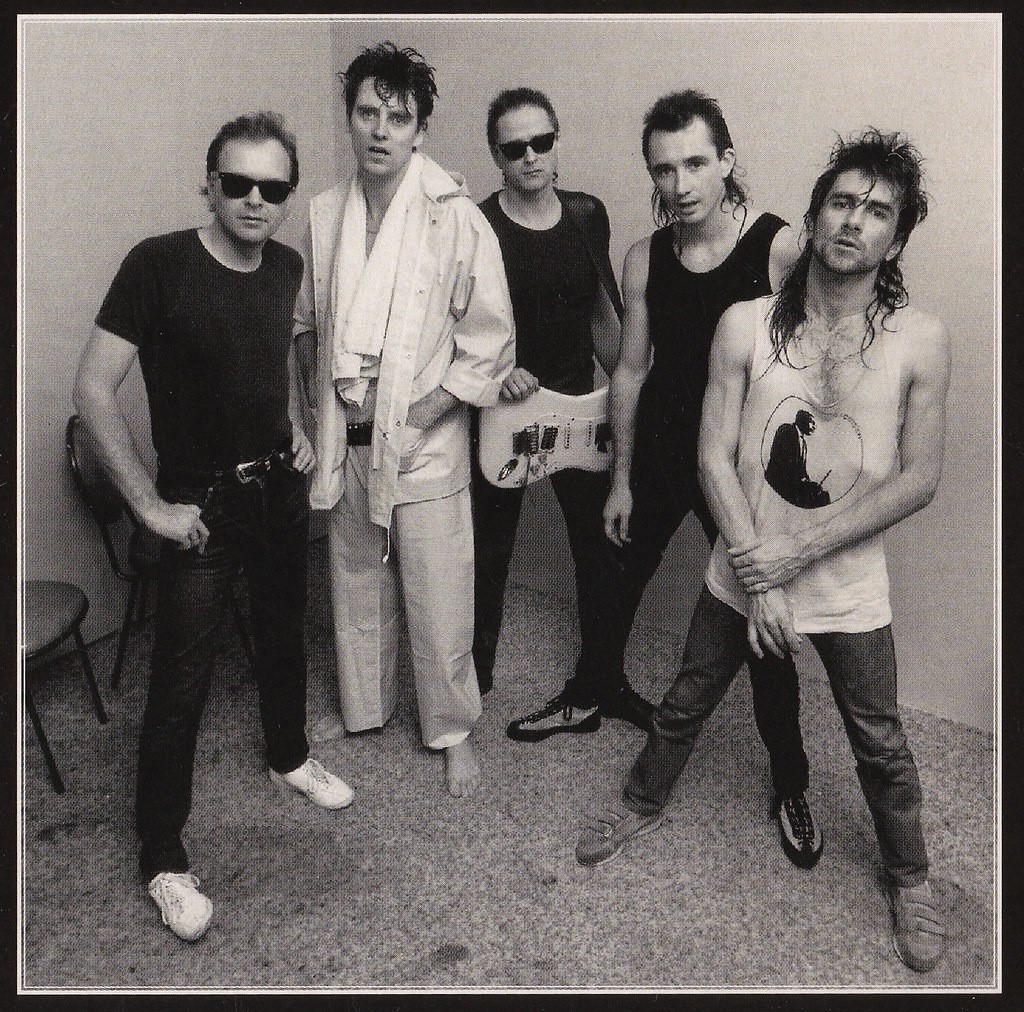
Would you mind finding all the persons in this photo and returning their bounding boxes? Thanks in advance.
[507,88,823,870]
[293,44,517,800]
[575,137,944,972]
[470,88,660,731]
[71,114,355,941]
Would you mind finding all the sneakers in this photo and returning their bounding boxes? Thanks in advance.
[268,764,355,810]
[148,870,213,940]
[883,880,944,973]
[772,793,823,868]
[576,798,662,866]
[507,697,601,742]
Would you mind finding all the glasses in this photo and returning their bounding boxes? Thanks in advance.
[218,171,293,204]
[498,131,557,162]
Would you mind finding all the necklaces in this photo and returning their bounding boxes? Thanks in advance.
[794,363,866,408]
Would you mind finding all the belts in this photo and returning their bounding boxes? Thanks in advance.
[345,422,373,446]
[161,441,294,485]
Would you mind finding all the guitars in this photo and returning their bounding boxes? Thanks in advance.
[476,385,616,489]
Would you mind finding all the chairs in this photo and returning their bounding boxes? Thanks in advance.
[23,578,109,793]
[67,414,256,690]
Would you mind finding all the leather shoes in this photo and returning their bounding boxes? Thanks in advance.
[601,685,659,732]
[478,673,494,696]
